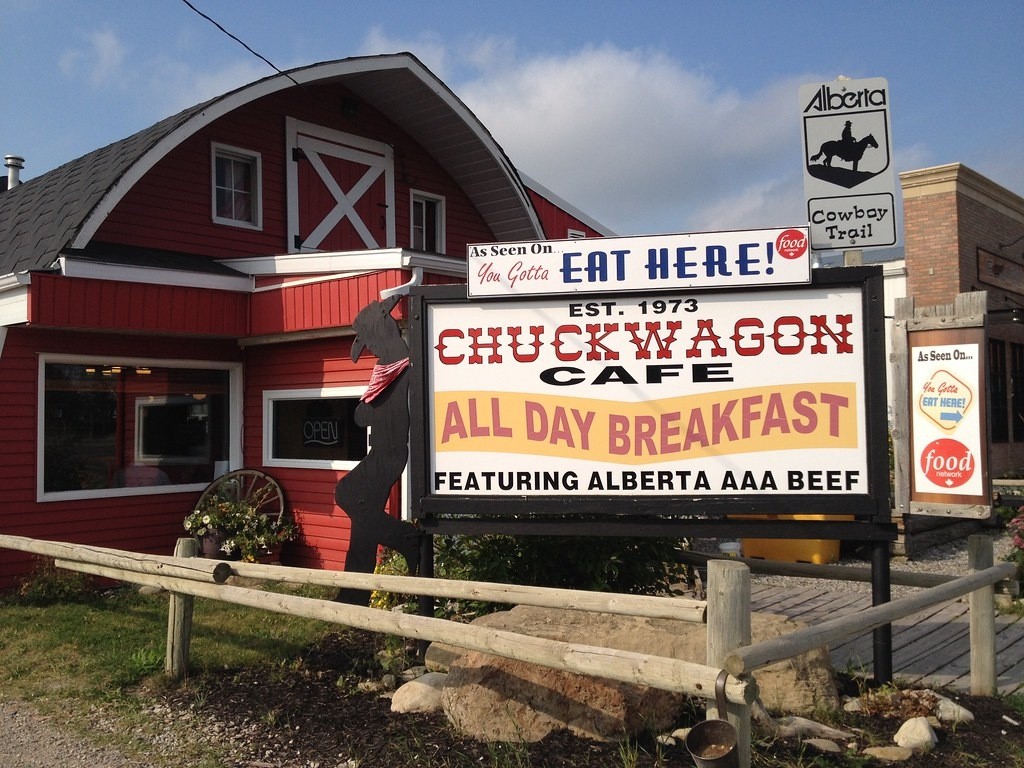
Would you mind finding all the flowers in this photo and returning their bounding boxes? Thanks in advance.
[182,471,300,564]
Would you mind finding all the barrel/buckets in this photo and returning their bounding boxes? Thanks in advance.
[685,720,739,768]
[719,542,741,558]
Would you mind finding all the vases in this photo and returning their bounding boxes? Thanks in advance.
[256,542,282,564]
[194,528,235,560]
